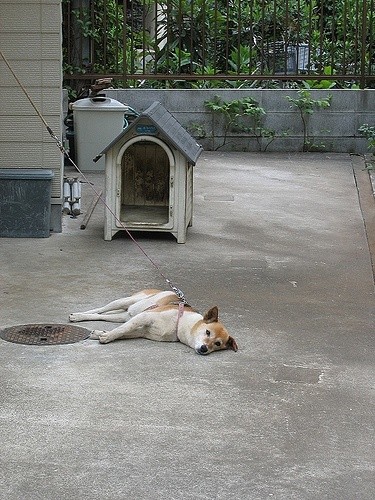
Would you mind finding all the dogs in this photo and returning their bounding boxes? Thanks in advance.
[68,289,238,356]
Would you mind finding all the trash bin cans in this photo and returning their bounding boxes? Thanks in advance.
[0,168,54,239]
[69,97,130,173]
